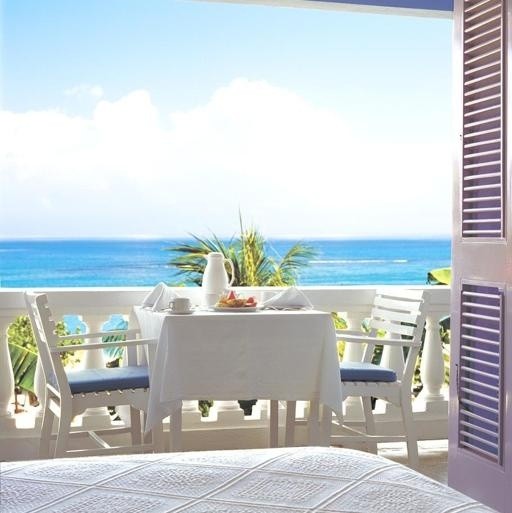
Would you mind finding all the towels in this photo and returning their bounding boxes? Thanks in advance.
[143,281,186,310]
[262,285,316,309]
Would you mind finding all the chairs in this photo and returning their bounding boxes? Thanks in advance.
[22,288,186,457]
[317,285,430,465]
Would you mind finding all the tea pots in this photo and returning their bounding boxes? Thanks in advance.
[201,252,235,306]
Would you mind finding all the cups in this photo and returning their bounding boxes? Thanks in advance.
[169,297,189,312]
[260,291,278,303]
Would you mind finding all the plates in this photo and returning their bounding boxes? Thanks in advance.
[209,304,264,312]
[164,309,196,315]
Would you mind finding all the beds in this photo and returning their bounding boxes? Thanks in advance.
[0,446,501,513]
